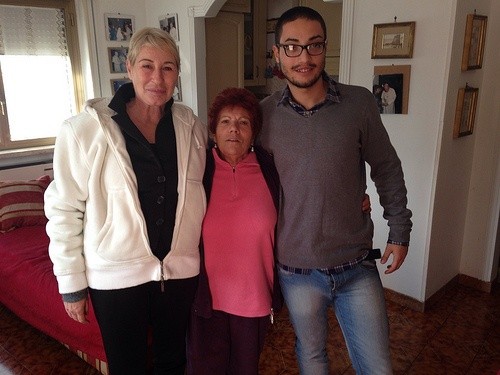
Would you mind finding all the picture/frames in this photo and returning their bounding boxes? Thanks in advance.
[103,11,135,42]
[110,77,131,96]
[108,47,130,73]
[370,22,415,59]
[371,65,415,115]
[453,86,479,139]
[461,13,489,72]
[159,14,183,102]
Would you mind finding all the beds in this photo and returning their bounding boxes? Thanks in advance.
[0,219,109,375]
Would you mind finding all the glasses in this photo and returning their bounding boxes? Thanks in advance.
[277,41,328,57]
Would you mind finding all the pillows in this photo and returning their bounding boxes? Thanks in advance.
[0,173,50,234]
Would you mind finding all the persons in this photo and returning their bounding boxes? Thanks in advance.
[106,17,183,106]
[183,89,374,374]
[254,6,413,374]
[371,79,397,113]
[42,26,211,375]
